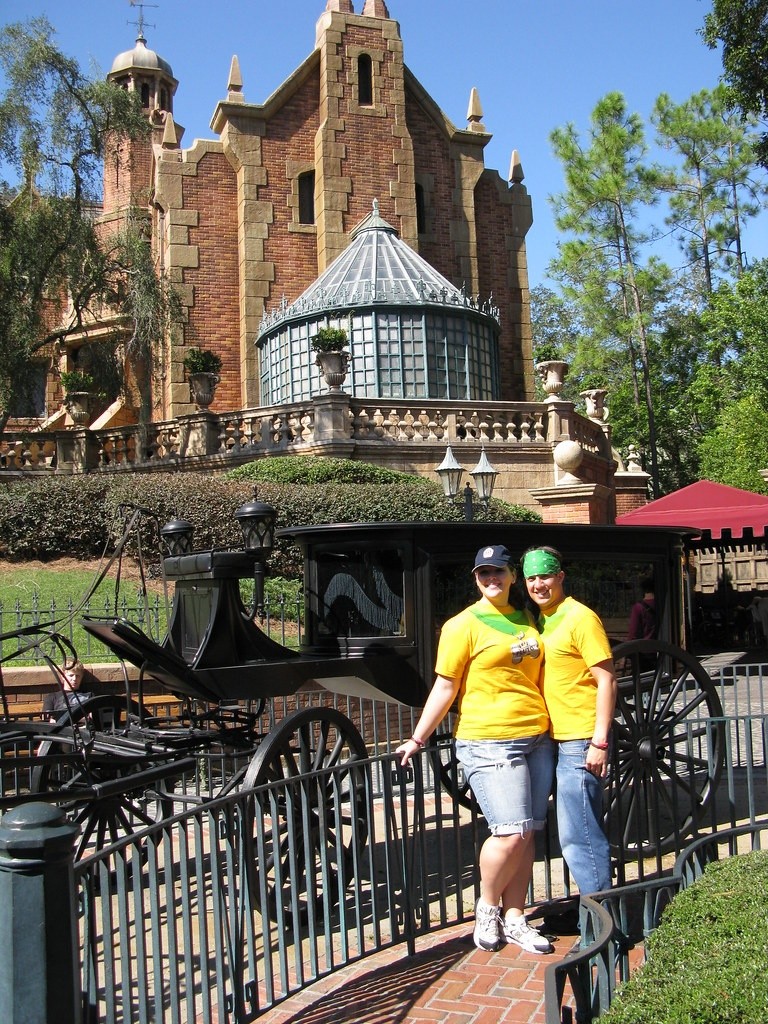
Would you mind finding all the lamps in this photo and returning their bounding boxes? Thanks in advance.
[156,485,277,625]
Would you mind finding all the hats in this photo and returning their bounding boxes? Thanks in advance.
[471,544,514,572]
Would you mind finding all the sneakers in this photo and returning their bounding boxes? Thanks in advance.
[473,896,503,952]
[498,917,555,954]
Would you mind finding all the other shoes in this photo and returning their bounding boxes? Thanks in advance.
[541,908,580,935]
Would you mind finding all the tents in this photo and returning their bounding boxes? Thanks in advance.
[615,480,768,539]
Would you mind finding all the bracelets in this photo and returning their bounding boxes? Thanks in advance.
[411,736,425,747]
[591,743,609,750]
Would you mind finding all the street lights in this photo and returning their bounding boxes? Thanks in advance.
[435,440,501,521]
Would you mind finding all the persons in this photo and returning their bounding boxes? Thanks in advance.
[628,579,657,670]
[397,545,556,953]
[42,659,95,754]
[747,597,761,623]
[519,546,617,895]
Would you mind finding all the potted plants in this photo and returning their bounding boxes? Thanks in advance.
[58,370,94,429]
[183,347,223,413]
[532,344,569,403]
[310,326,353,393]
[579,373,608,424]
